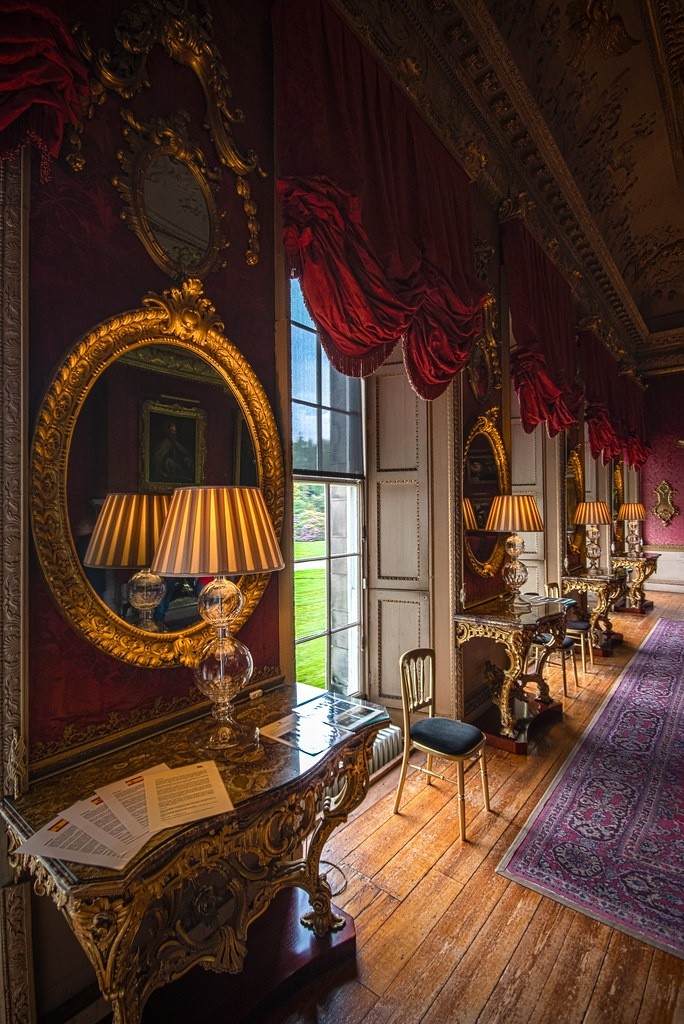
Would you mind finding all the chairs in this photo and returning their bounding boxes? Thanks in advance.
[394,649,490,841]
[524,582,594,696]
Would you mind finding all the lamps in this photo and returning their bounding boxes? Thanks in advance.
[572,502,611,572]
[84,492,174,633]
[486,495,545,607]
[151,486,285,761]
[617,503,647,555]
[463,498,478,529]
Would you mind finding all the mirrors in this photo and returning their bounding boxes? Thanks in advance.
[29,273,285,669]
[613,465,623,540]
[463,404,511,577]
[567,452,585,555]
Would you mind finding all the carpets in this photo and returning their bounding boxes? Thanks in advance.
[495,618,684,960]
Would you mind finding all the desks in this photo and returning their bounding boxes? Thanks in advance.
[454,553,663,738]
[0,682,392,1024]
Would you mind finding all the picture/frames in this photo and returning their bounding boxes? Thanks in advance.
[111,105,231,282]
[467,330,494,404]
[467,450,499,484]
[139,399,208,492]
